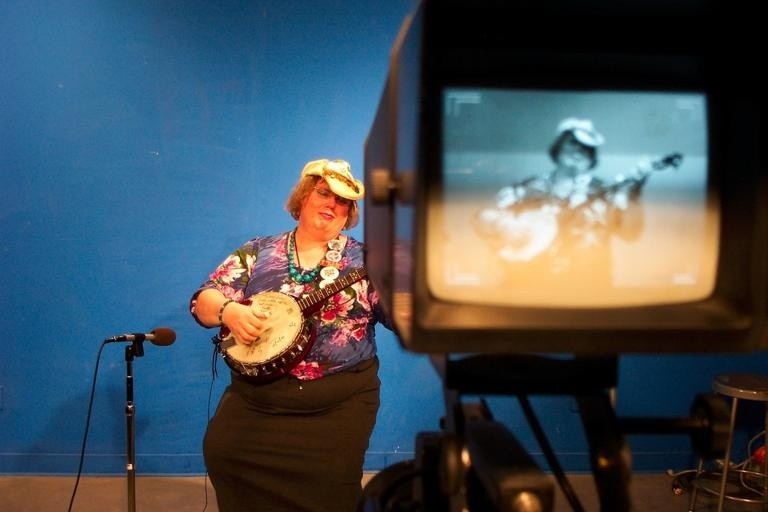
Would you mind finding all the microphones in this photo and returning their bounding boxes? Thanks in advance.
[106,327,176,345]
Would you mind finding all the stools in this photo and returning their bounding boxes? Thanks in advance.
[688,371,768,512]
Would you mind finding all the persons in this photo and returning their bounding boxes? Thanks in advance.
[481,118,645,278]
[190,159,392,512]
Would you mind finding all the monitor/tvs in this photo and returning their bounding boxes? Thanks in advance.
[425,85,724,310]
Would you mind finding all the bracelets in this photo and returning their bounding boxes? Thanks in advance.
[218,299,234,323]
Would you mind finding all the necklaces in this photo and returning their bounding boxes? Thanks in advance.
[288,227,321,284]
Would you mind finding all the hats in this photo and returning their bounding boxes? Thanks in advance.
[301,159,365,200]
[555,117,605,146]
